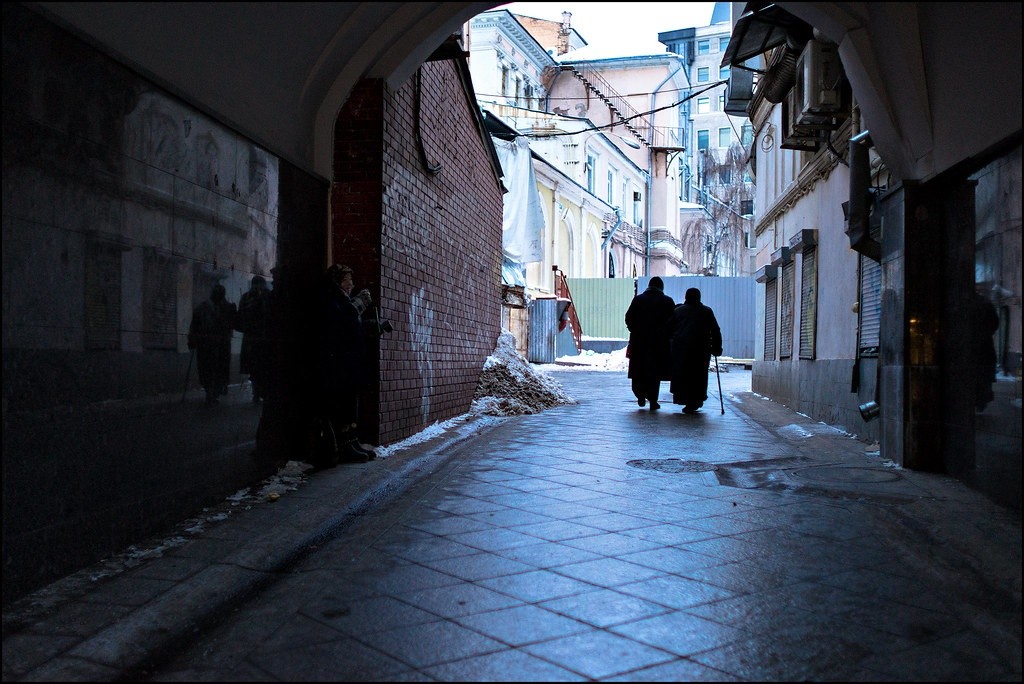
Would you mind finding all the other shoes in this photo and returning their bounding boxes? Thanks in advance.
[638,398,646,406]
[650,403,660,411]
[682,405,699,412]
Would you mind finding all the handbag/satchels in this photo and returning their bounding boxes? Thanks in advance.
[308,417,337,469]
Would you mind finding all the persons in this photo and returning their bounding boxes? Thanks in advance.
[187,277,274,405]
[317,262,377,462]
[625,276,722,413]
[919,261,999,473]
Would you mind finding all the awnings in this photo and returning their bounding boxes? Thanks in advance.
[720,1,811,73]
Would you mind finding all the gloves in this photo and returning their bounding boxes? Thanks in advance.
[357,288,372,306]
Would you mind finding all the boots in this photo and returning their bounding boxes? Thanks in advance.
[334,424,375,462]
